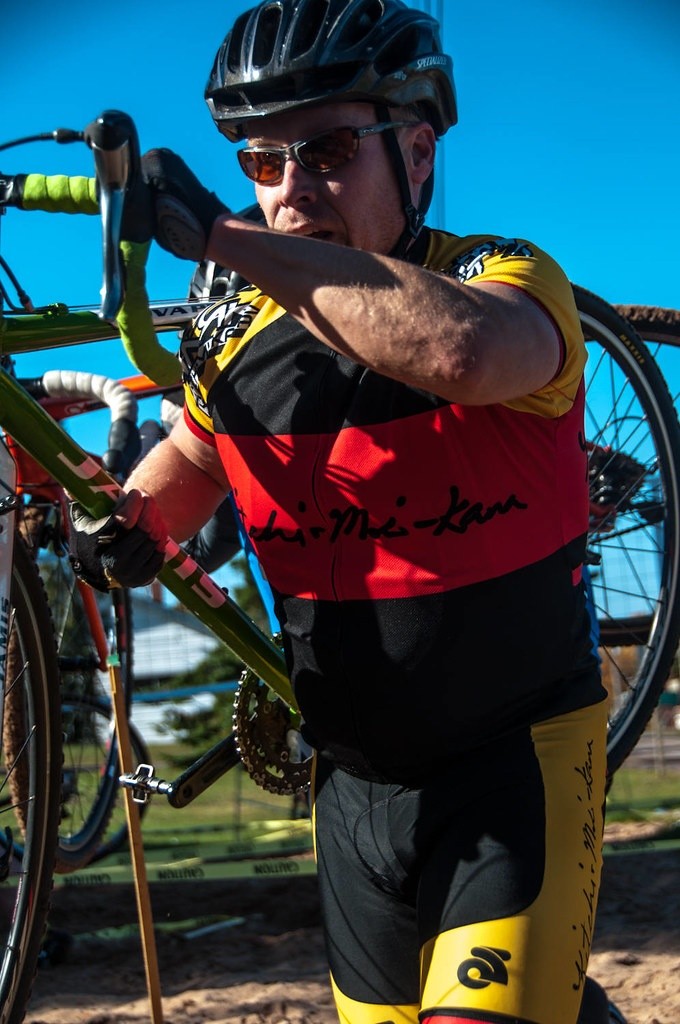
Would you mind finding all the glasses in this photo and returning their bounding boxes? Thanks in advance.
[236,120,421,185]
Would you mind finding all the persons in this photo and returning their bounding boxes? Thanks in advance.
[66,0,610,1024]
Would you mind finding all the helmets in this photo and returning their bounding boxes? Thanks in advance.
[205,0,458,136]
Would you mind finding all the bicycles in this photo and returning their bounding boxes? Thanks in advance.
[1,109,680,1024]
